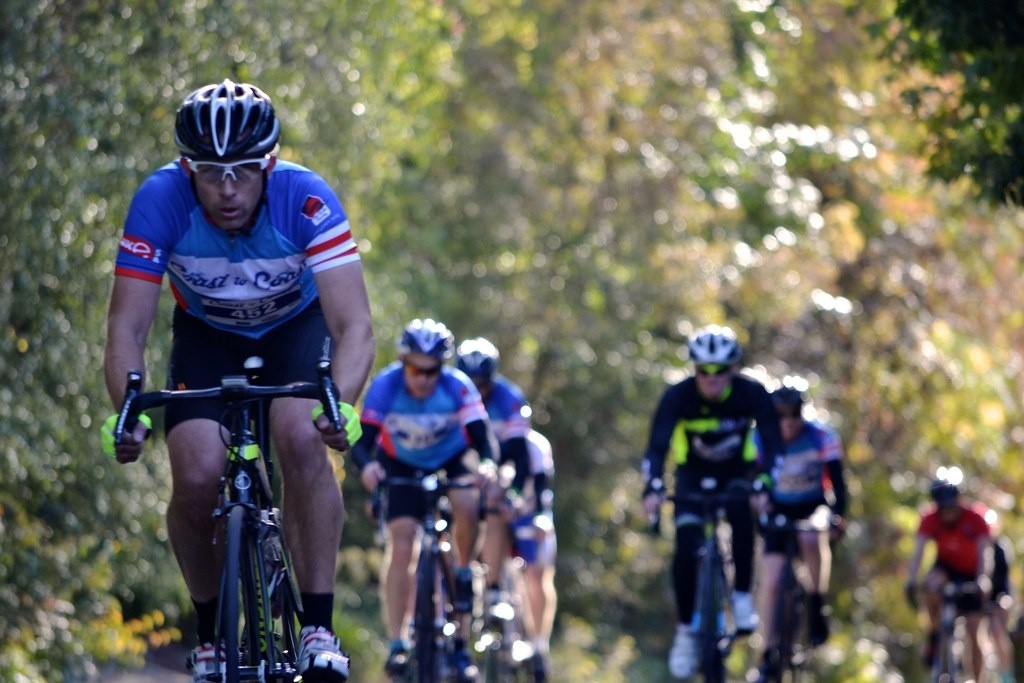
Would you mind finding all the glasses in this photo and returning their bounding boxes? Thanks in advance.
[185,155,270,186]
[403,357,442,378]
[696,364,731,377]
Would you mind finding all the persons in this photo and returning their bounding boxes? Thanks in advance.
[752,380,850,683]
[642,324,784,678]
[100,79,377,683]
[456,338,559,683]
[904,468,1016,683]
[351,318,501,683]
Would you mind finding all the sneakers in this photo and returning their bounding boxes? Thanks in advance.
[192,641,226,683]
[296,625,351,683]
[448,567,475,613]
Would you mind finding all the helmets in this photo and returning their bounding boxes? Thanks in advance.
[688,325,743,365]
[929,480,960,501]
[173,78,280,160]
[398,316,455,363]
[455,335,500,381]
[772,387,803,417]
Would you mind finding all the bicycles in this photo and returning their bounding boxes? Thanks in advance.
[372,468,533,683]
[646,480,767,683]
[757,507,837,683]
[114,356,342,683]
[910,578,993,683]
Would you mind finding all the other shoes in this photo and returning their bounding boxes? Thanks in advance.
[668,625,699,677]
[523,651,546,683]
[451,649,476,678]
[919,631,941,670]
[384,647,411,674]
[728,590,762,631]
[804,592,829,645]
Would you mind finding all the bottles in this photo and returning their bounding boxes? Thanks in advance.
[422,518,438,552]
[260,509,284,537]
